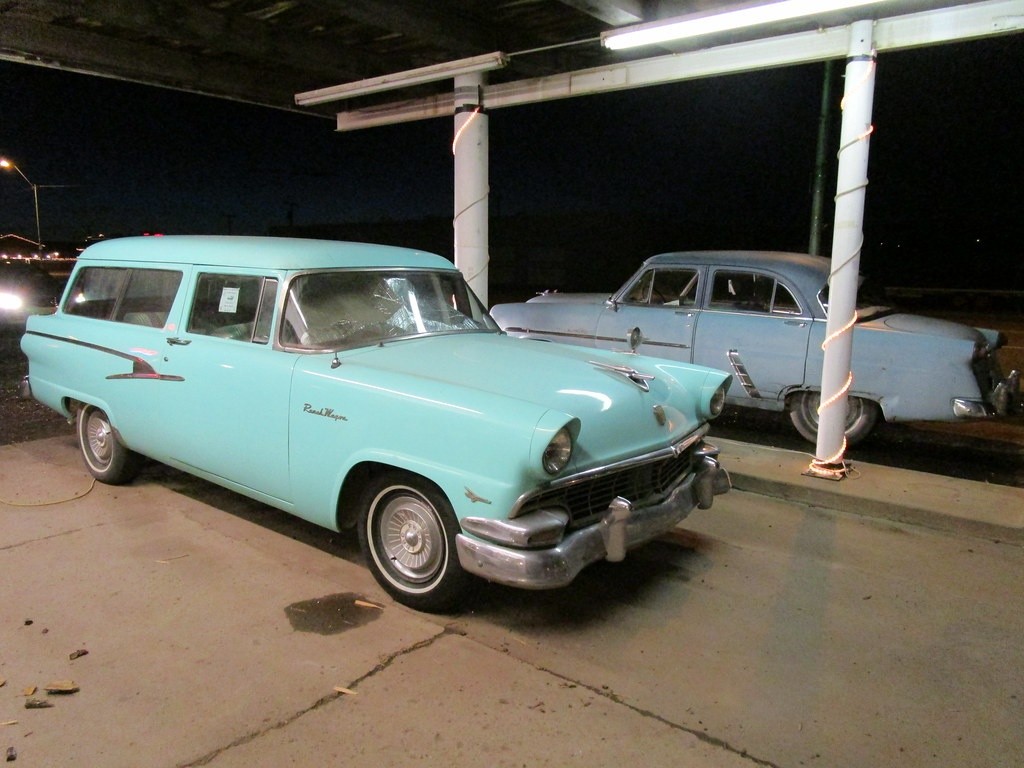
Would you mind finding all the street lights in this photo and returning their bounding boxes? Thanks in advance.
[1,159,45,275]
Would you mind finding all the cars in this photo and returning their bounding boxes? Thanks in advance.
[489,249,1021,446]
[0,261,88,334]
[19,235,734,613]
